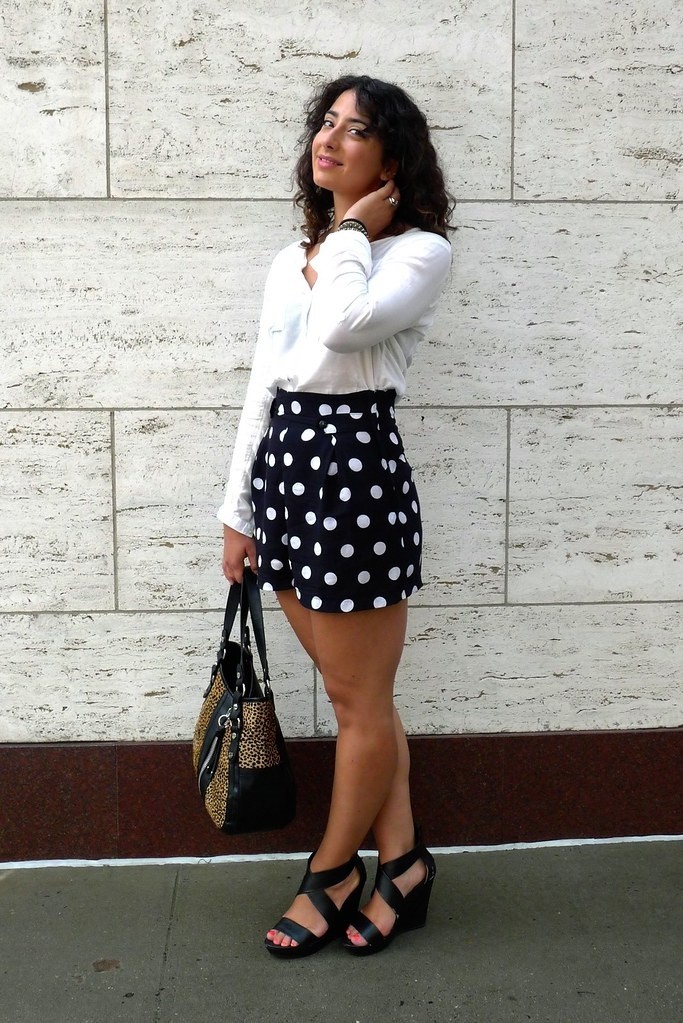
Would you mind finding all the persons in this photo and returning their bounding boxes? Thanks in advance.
[216,74,457,961]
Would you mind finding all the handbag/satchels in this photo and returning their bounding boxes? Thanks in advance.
[192,565,296,835]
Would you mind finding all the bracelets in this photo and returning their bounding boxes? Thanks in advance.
[337,218,371,239]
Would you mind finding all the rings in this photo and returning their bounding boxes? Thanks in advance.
[388,196,399,206]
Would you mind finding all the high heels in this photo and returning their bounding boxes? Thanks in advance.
[341,837,437,955]
[263,851,366,959]
